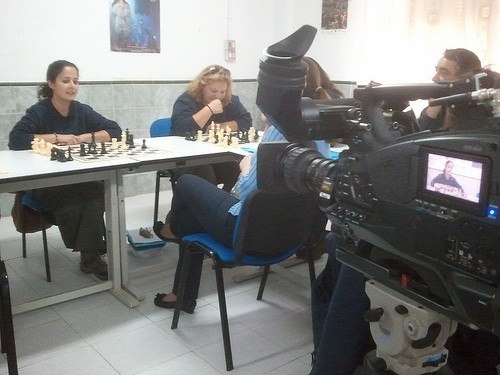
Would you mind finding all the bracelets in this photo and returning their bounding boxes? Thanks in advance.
[54,133,57,144]
[206,104,213,114]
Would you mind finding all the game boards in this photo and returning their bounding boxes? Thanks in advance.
[185,130,264,147]
[57,139,167,164]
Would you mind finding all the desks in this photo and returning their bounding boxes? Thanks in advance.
[229,140,349,290]
[0,128,264,315]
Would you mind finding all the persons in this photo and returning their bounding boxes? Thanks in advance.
[169,65,252,192]
[417,48,481,131]
[9,59,122,281]
[309,236,373,375]
[431,160,465,195]
[153,56,350,314]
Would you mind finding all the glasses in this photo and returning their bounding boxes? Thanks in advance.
[210,67,230,76]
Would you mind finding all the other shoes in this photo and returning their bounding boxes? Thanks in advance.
[74,234,107,254]
[153,221,180,243]
[154,293,196,314]
[81,255,108,280]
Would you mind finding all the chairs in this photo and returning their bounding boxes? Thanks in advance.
[150,118,179,224]
[20,190,106,282]
[170,140,319,371]
